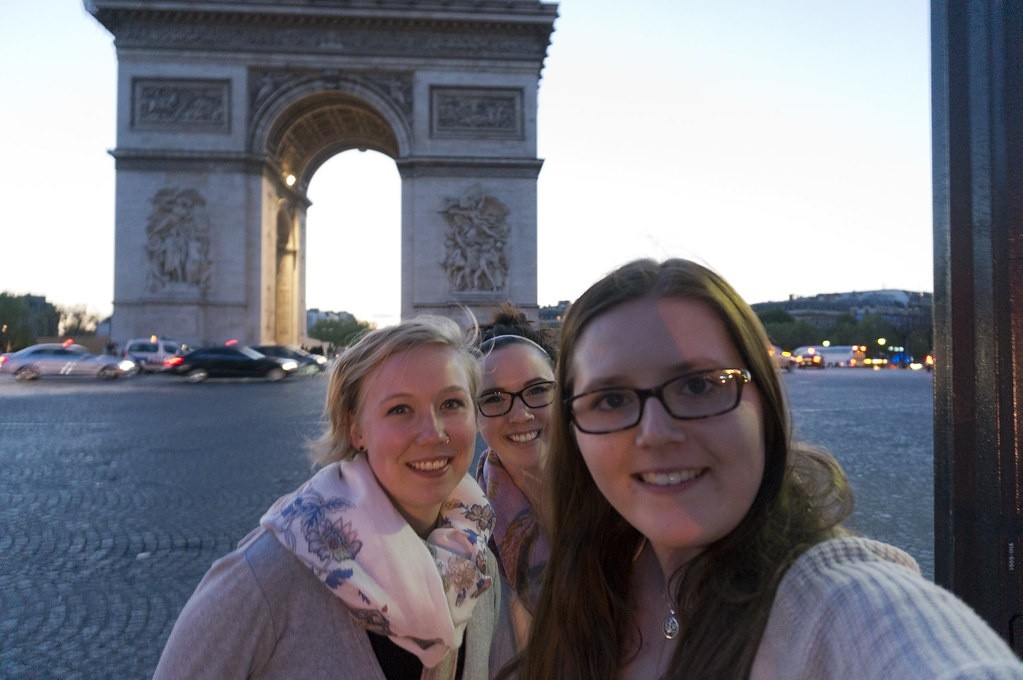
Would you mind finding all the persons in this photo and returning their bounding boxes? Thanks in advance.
[466,303,565,665]
[498,256,1023,680]
[147,311,499,680]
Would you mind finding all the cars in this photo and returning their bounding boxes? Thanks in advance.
[250,343,331,374]
[164,338,300,385]
[0,339,141,380]
[770,340,936,373]
[123,336,199,373]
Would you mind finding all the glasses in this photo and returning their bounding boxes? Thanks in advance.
[562,368,751,436]
[478,381,559,419]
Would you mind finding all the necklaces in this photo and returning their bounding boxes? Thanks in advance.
[657,565,693,640]
